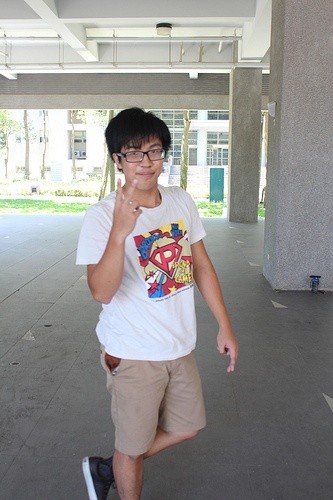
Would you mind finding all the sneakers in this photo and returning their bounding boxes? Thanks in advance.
[82,456,116,500]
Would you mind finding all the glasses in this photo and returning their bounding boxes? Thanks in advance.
[117,148,167,163]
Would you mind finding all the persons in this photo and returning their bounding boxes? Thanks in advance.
[74,106,240,500]
